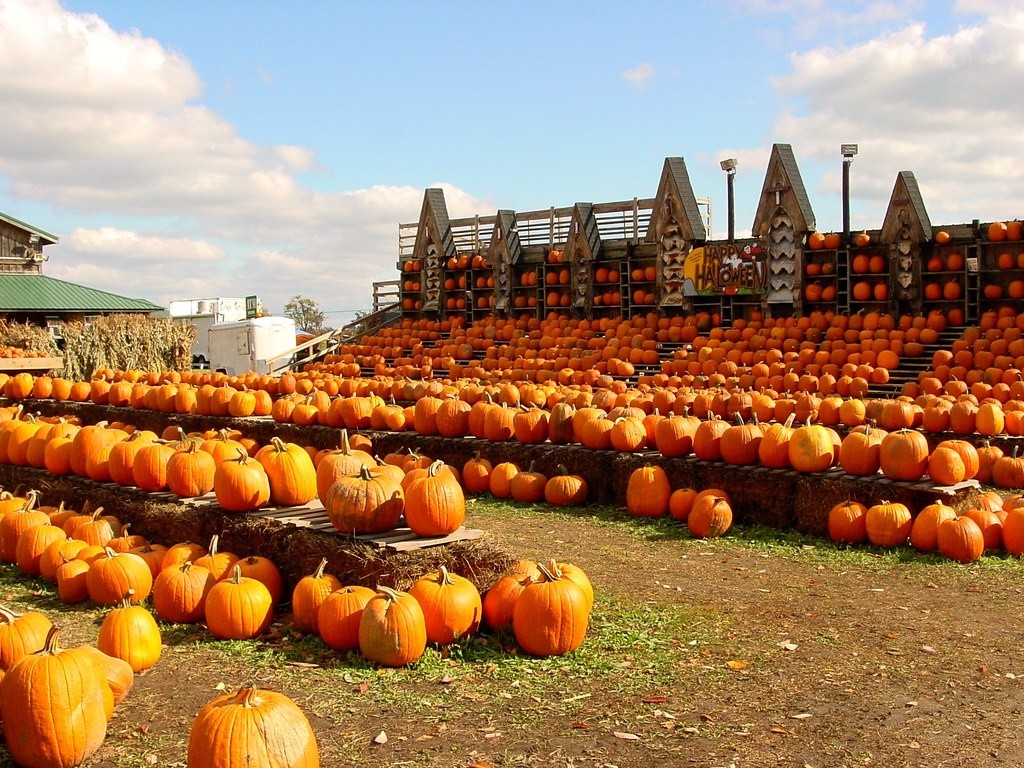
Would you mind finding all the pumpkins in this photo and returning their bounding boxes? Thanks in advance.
[0,218,1024,768]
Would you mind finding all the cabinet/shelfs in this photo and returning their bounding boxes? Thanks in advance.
[511,264,542,318]
[627,256,660,321]
[976,238,1024,325]
[399,270,425,320]
[802,248,839,314]
[592,260,625,321]
[542,261,571,320]
[846,244,892,316]
[919,244,969,322]
[443,269,469,322]
[471,267,495,322]
